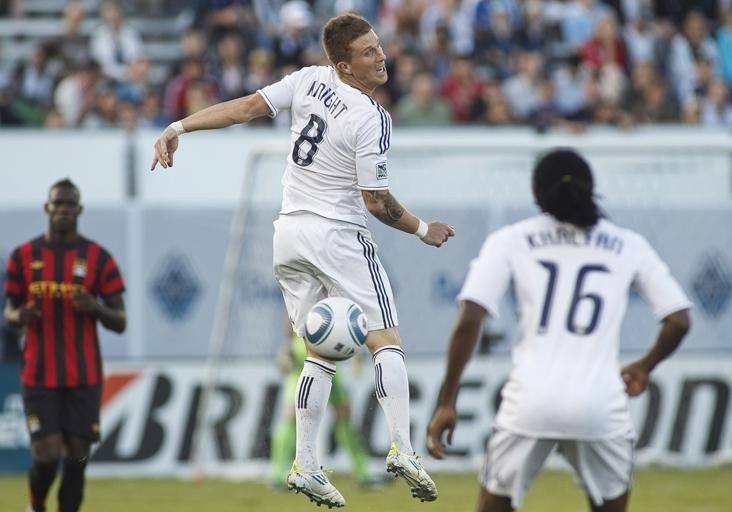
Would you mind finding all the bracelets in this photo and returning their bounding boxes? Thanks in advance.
[412,218,431,239]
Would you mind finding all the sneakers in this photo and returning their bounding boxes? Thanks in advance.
[287,461,346,510]
[385,443,438,503]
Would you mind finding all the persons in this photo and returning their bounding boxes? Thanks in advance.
[1,2,731,134]
[422,142,698,512]
[0,177,129,512]
[257,316,402,495]
[144,9,457,509]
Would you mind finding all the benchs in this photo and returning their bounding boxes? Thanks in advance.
[0,0,199,125]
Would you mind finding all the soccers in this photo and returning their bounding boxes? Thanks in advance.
[305,297,369,361]
